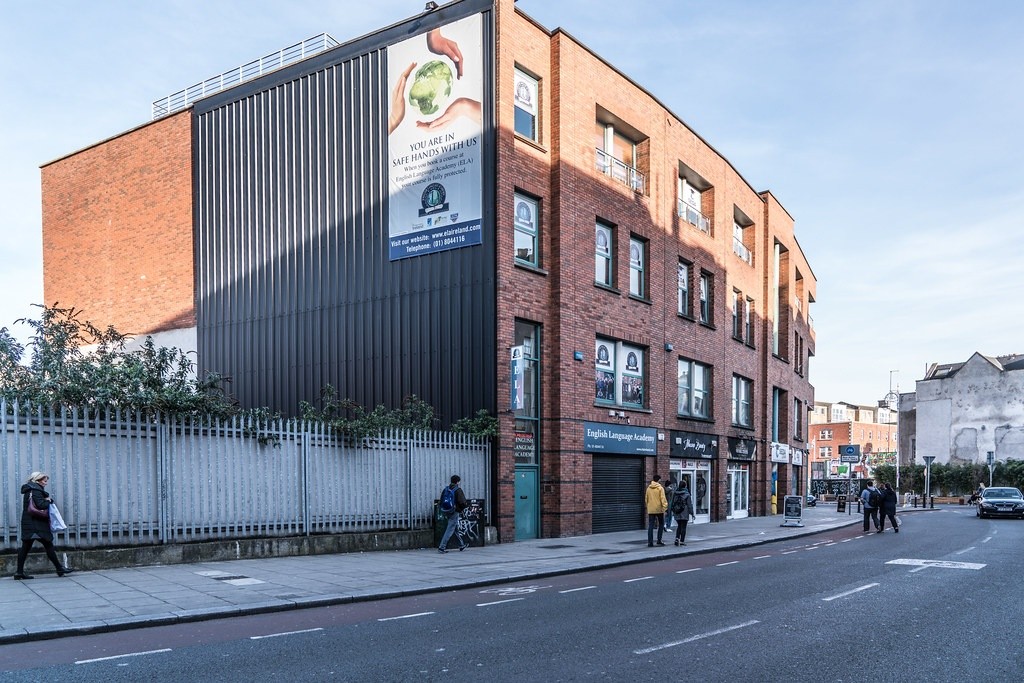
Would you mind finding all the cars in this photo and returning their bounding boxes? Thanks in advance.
[807,495,816,506]
[976,487,1024,519]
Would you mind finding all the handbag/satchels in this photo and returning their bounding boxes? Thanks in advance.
[27,502,48,517]
[49,503,67,532]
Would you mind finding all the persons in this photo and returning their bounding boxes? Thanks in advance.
[437,475,469,554]
[388,27,482,134]
[967,482,985,507]
[645,475,695,547]
[860,481,899,534]
[14,471,73,580]
[596,376,642,404]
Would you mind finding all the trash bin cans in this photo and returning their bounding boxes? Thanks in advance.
[837,495,846,512]
[959,498,964,504]
[435,497,489,547]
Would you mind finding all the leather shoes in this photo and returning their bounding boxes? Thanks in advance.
[57,566,74,577]
[14,572,34,580]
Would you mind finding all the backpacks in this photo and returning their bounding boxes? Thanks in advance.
[440,486,459,515]
[671,490,689,515]
[866,488,881,507]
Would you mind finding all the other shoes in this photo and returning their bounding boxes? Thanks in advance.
[877,526,881,531]
[667,529,673,532]
[664,524,667,532]
[648,543,653,547]
[460,543,469,551]
[877,529,883,533]
[675,539,679,546]
[680,543,687,547]
[895,527,898,533]
[861,531,869,534]
[657,540,665,546]
[438,548,450,554]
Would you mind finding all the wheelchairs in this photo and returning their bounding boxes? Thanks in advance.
[968,493,977,506]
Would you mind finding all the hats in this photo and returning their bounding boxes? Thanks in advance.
[28,472,46,483]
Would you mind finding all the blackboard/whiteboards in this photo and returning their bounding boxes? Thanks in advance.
[783,495,802,520]
